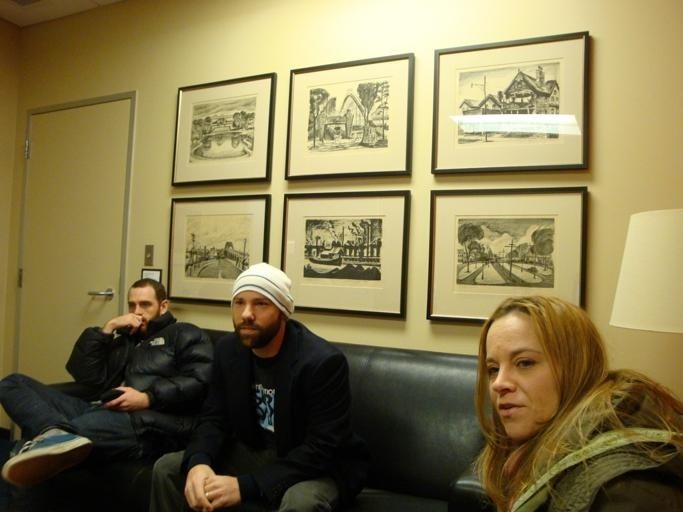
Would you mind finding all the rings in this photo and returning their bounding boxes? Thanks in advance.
[205,492,209,499]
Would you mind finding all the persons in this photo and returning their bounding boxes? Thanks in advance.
[149,261,353,511]
[0,277,214,512]
[470,295,683,512]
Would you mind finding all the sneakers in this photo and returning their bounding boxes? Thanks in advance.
[3,429,97,485]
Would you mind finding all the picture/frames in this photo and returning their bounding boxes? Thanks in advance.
[431,30,590,175]
[141,268,162,285]
[171,72,277,188]
[426,185,588,324]
[284,53,415,181]
[167,194,271,307]
[280,190,410,321]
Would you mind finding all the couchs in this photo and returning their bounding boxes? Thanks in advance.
[21,330,509,512]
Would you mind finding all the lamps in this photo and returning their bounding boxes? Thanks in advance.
[609,209,683,333]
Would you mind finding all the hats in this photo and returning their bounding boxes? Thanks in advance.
[228,261,295,317]
